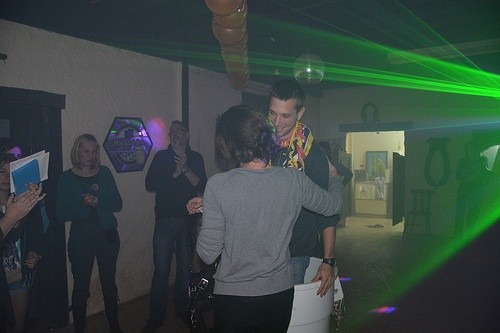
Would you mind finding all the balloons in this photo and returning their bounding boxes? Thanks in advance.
[205,0,246,45]
[218,35,249,89]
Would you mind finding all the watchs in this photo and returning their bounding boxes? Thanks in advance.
[323,258,337,267]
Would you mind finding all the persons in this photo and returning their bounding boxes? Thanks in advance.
[196,105,346,333]
[318,141,354,187]
[186,79,340,297]
[142,120,207,333]
[56,134,122,333]
[0,153,55,333]
[0,182,46,241]
[453,140,486,238]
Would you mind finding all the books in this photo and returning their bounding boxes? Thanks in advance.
[10,150,50,197]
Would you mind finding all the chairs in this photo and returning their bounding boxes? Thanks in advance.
[401,189,435,240]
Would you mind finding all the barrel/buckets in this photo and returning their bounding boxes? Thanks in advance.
[287,256,338,333]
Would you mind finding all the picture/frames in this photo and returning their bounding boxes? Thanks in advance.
[365,150,388,181]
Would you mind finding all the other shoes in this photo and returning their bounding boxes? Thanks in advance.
[175,304,189,324]
[142,318,164,333]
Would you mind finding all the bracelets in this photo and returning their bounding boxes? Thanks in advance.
[184,168,189,173]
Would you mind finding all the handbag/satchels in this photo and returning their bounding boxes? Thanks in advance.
[184,256,222,333]
